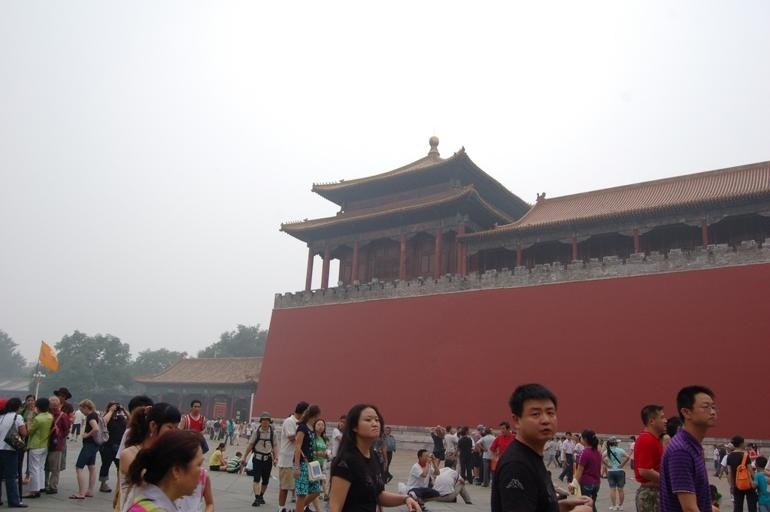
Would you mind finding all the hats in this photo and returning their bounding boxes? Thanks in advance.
[52,387,72,400]
[607,436,622,444]
[260,411,272,423]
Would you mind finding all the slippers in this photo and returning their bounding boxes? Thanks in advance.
[69,494,84,499]
[84,493,93,498]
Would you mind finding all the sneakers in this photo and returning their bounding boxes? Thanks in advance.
[252,501,260,507]
[99,486,112,492]
[260,499,265,504]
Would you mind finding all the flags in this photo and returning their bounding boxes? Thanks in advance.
[39,340,59,372]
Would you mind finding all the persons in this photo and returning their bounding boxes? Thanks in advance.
[206,418,241,445]
[561,436,566,446]
[382,425,396,484]
[430,427,445,460]
[659,385,718,512]
[748,456,770,512]
[277,400,310,511]
[568,429,602,512]
[719,443,737,512]
[373,428,388,473]
[54,386,75,427]
[242,420,247,438]
[175,467,215,512]
[727,435,759,512]
[292,404,323,511]
[178,399,206,433]
[99,400,130,493]
[245,424,253,442]
[21,398,54,499]
[69,399,100,499]
[69,404,84,442]
[250,419,258,442]
[747,443,760,471]
[327,404,422,512]
[490,421,514,458]
[454,425,463,448]
[312,419,331,501]
[573,437,585,469]
[633,404,667,512]
[443,428,459,460]
[226,452,246,475]
[559,432,574,482]
[244,448,258,476]
[331,415,347,458]
[574,434,580,449]
[407,449,440,505]
[629,435,636,470]
[660,433,672,448]
[710,483,722,512]
[476,427,496,488]
[471,425,485,481]
[122,430,204,512]
[462,427,477,477]
[112,402,182,511]
[555,437,562,468]
[718,444,726,468]
[208,443,228,471]
[39,398,70,495]
[442,425,452,450]
[491,383,593,512]
[433,456,473,504]
[601,437,630,512]
[0,398,29,508]
[457,431,473,484]
[19,394,37,483]
[544,438,558,469]
[712,444,720,477]
[241,412,278,506]
[666,416,683,438]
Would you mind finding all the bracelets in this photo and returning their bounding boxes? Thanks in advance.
[403,494,411,504]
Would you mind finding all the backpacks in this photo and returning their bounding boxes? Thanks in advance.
[90,415,110,446]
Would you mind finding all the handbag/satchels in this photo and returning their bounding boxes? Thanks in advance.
[301,439,322,482]
[4,414,26,449]
[48,412,64,449]
